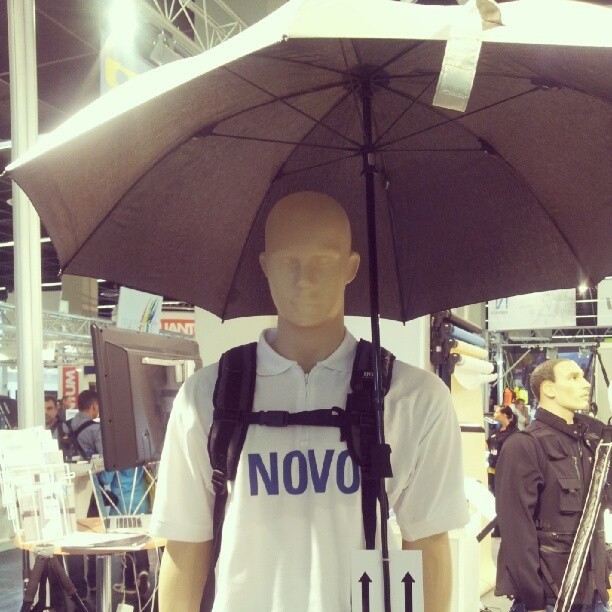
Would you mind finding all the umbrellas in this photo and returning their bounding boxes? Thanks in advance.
[3,2,611,478]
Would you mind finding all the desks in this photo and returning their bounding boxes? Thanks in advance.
[12,515,166,611]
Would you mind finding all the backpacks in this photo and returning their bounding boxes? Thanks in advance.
[59,419,99,462]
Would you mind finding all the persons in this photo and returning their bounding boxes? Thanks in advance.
[53,388,103,602]
[43,395,61,436]
[490,358,612,610]
[487,404,520,538]
[513,398,530,430]
[153,188,459,612]
